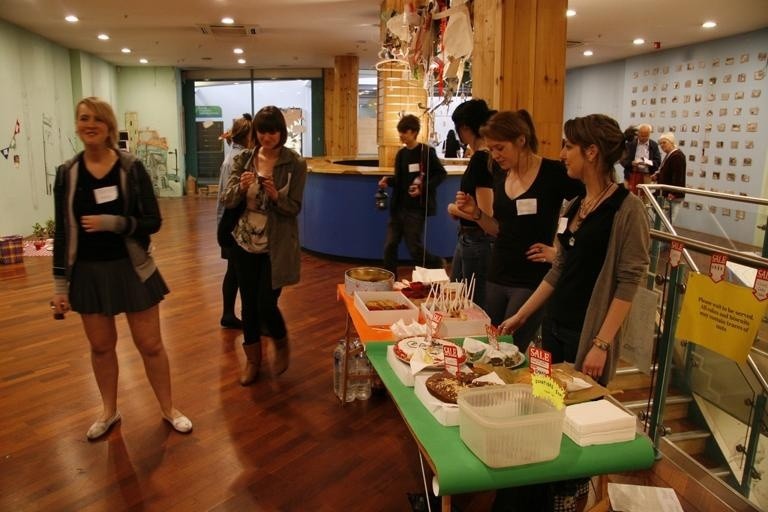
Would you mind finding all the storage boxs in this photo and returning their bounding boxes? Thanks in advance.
[0,234,24,265]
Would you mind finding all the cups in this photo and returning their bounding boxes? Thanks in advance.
[33,239,54,250]
[257,177,269,184]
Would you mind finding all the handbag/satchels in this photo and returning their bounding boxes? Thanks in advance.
[217,143,259,250]
[460,235,500,271]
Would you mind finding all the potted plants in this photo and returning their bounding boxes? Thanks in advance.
[31,223,48,252]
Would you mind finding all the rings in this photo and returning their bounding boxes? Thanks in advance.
[498,326,503,329]
[587,369,593,376]
[542,258,546,262]
[503,326,508,330]
[538,247,544,253]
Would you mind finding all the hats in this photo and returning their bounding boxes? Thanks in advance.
[658,130,676,148]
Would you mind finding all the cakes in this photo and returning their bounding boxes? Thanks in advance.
[463,336,520,368]
[425,371,506,405]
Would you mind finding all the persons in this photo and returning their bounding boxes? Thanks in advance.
[619,124,661,213]
[443,130,461,158]
[455,108,586,354]
[50,97,193,440]
[215,115,257,329]
[649,132,685,253]
[378,114,447,282]
[220,105,306,386]
[496,113,650,388]
[446,99,496,314]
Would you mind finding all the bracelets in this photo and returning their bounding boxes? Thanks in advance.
[473,207,481,220]
[592,336,611,352]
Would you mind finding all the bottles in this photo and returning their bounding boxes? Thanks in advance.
[185,177,195,194]
[332,338,387,401]
[374,188,390,210]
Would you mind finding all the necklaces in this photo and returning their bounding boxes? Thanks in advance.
[569,181,613,220]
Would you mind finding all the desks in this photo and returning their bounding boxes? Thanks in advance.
[337,282,661,512]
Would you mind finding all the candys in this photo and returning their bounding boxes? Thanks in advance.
[423,303,473,320]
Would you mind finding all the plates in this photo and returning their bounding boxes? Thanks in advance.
[468,351,526,370]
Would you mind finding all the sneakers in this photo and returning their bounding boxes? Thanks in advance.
[86,409,122,441]
[219,314,243,328]
[159,407,193,434]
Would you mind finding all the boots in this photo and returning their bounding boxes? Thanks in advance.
[238,340,262,386]
[270,333,290,376]
